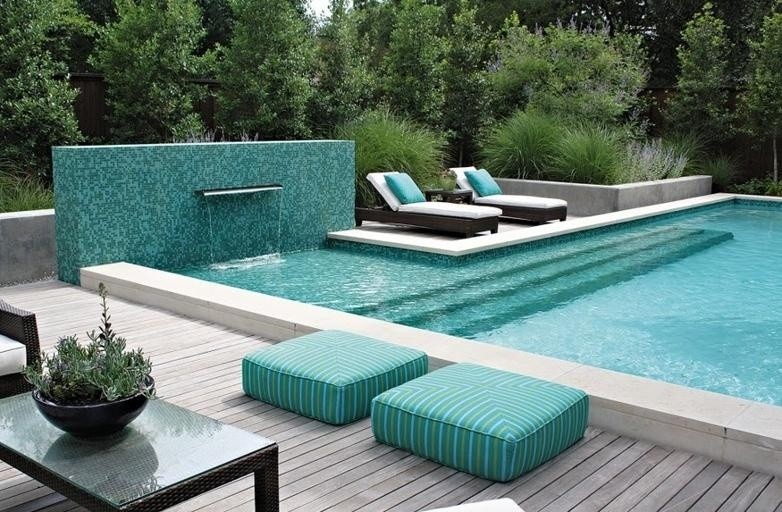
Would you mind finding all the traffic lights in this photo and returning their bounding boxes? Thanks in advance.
[385,173,424,205]
[464,167,502,197]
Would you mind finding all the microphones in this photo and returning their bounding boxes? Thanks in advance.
[370,359,590,483]
[241,328,430,428]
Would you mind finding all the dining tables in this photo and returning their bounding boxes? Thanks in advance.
[22,285,155,434]
[437,169,458,191]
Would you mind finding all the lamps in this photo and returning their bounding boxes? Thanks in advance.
[356,167,503,237]
[447,161,567,225]
[0,294,43,396]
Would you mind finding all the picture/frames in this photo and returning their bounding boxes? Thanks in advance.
[0,376,279,512]
[424,187,473,205]
